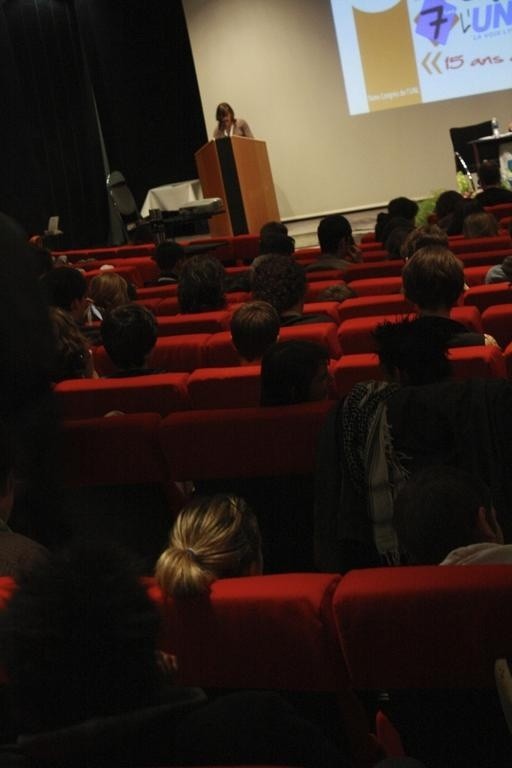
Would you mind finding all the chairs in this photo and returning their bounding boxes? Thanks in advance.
[450,117,499,188]
[105,171,166,248]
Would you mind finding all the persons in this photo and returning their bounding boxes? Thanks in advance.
[0,180,512,768]
[208,100,252,137]
